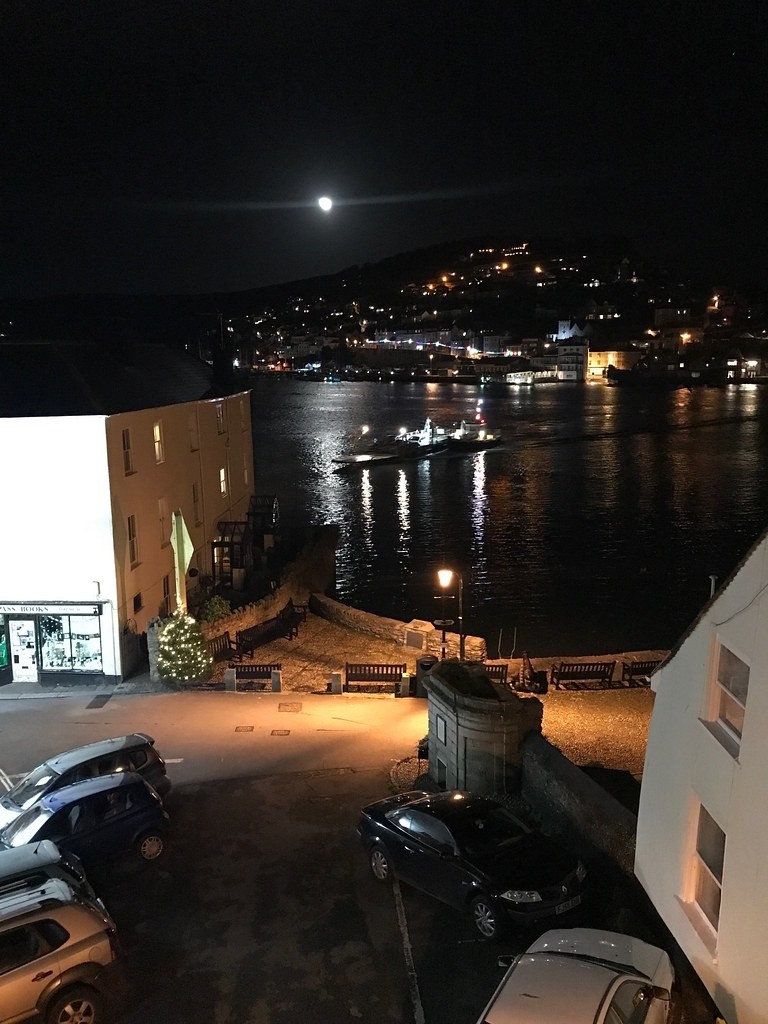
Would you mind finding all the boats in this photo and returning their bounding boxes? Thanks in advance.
[449,399,501,449]
[332,417,456,463]
[483,381,510,399]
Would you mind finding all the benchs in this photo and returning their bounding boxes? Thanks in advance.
[228,664,282,680]
[206,597,307,664]
[346,661,406,691]
[550,661,616,690]
[484,664,508,684]
[622,660,663,687]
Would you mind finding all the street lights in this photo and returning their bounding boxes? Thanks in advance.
[710,575,717,598]
[428,353,434,374]
[437,568,465,660]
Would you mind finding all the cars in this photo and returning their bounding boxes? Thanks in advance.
[0,878,125,1024]
[476,928,676,1024]
[0,839,95,899]
[0,733,171,833]
[0,773,170,885]
[357,790,593,938]
